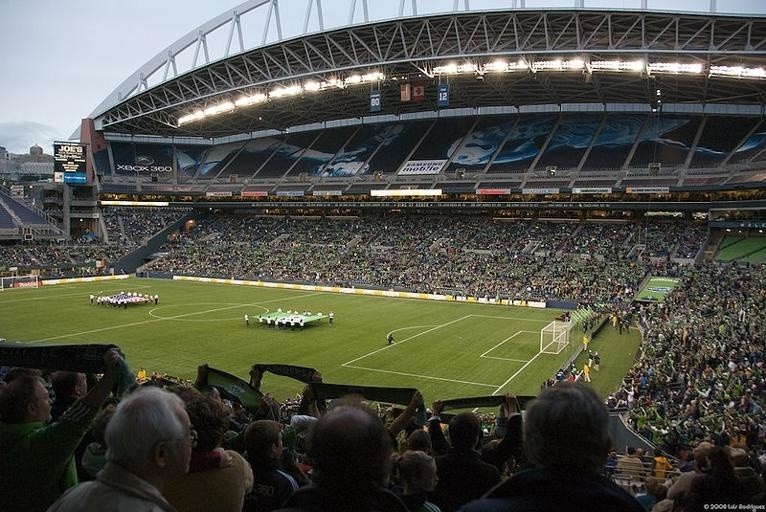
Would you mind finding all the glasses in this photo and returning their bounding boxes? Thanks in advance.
[162,429,199,447]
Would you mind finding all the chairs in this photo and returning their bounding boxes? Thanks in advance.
[0,105,766,324]
[595,326,766,511]
